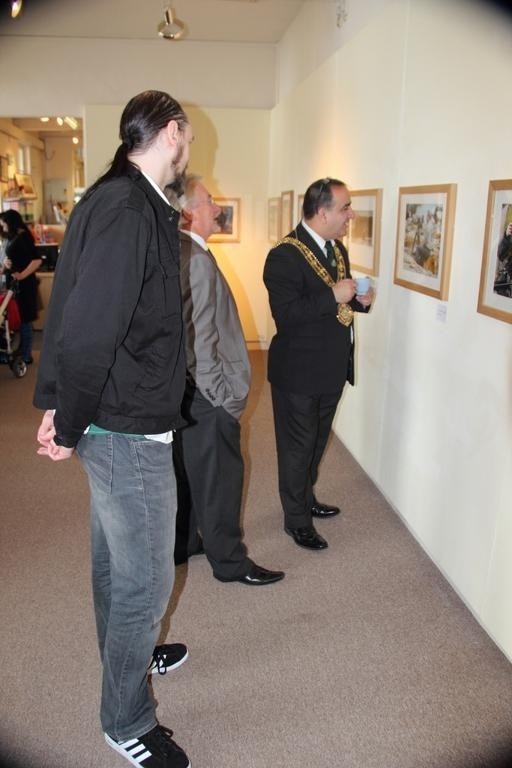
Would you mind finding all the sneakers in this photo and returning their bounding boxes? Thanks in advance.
[103,723,191,768]
[150,642,189,675]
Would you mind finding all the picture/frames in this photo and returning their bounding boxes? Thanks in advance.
[267,198,281,245]
[280,190,293,241]
[296,194,307,223]
[205,197,242,244]
[339,189,384,277]
[477,179,512,326]
[394,183,459,301]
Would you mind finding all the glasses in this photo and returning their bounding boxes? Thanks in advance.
[315,176,332,215]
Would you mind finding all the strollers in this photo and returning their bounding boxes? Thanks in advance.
[0,279,27,378]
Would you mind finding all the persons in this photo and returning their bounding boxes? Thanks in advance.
[263,176,373,550]
[31,92,194,768]
[495,220,511,299]
[0,208,42,366]
[412,209,439,279]
[174,173,285,587]
[0,226,7,276]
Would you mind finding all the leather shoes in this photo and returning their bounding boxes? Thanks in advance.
[283,524,328,551]
[212,561,286,586]
[310,501,340,518]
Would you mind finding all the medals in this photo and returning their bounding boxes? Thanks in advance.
[274,236,355,327]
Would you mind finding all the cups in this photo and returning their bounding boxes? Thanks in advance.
[353,276,371,296]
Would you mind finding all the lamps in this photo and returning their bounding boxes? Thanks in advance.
[0,0,22,18]
